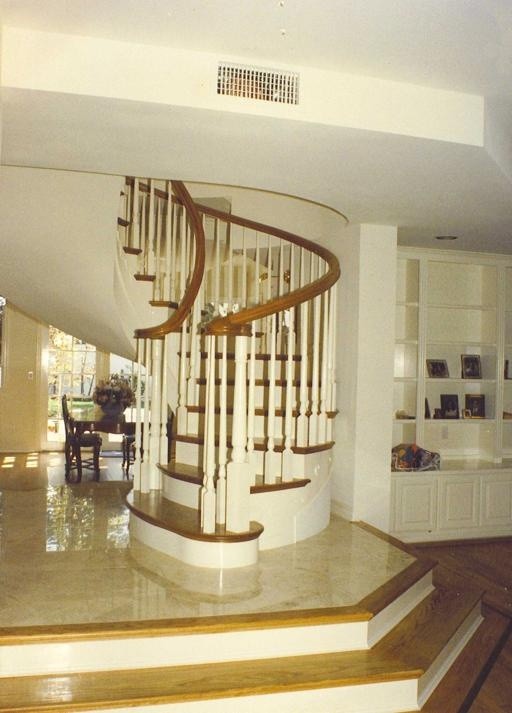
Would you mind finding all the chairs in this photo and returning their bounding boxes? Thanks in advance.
[61,394,173,484]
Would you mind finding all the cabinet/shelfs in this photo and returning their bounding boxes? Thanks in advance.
[389,246,512,544]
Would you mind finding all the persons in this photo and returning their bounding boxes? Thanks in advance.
[435,409,442,417]
[469,398,481,416]
[444,399,457,416]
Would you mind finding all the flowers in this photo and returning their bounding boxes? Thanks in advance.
[93,372,137,408]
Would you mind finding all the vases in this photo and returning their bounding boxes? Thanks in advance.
[100,403,126,415]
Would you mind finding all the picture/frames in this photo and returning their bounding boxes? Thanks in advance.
[461,354,482,379]
[440,394,459,419]
[465,395,485,419]
[426,360,449,377]
